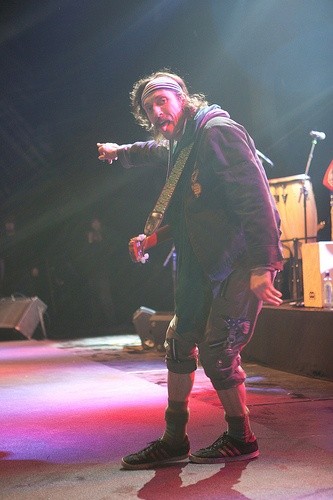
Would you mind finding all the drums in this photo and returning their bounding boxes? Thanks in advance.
[281,173,317,260]
[269,177,291,259]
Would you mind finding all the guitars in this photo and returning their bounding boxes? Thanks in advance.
[128,222,172,265]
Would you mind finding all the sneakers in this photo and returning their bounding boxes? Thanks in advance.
[189,430,260,464]
[120,433,192,471]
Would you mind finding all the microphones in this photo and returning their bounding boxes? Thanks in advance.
[310,130,327,141]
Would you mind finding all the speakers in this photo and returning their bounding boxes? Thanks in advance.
[132,307,175,349]
[0,296,47,339]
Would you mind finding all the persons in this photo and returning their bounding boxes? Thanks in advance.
[95,71,285,470]
[2,215,28,295]
[86,218,118,329]
